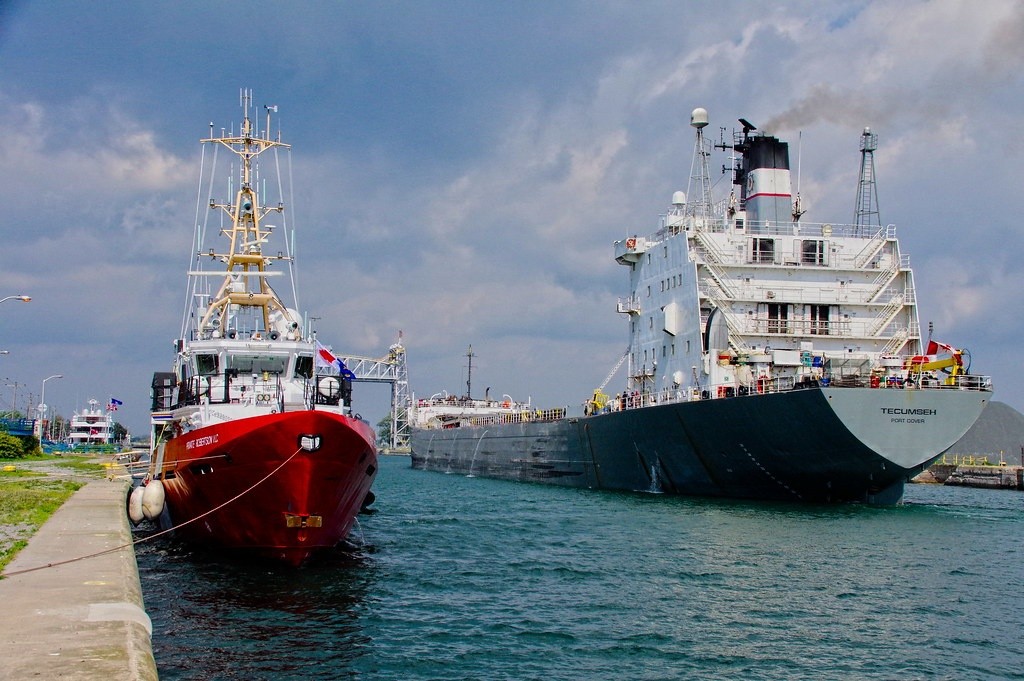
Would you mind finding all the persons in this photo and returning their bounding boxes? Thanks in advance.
[615,390,640,410]
[583,397,590,415]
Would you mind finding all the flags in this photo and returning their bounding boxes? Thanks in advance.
[111,397,123,404]
[316,339,337,369]
[90,428,98,434]
[923,338,963,365]
[107,403,117,411]
[337,358,357,380]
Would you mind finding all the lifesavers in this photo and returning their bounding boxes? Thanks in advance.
[738,386,748,396]
[725,387,737,398]
[700,389,713,400]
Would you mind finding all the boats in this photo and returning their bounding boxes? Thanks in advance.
[68,395,130,453]
[117,87,381,565]
[405,106,994,507]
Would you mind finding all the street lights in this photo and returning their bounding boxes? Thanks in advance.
[38,375,63,447]
